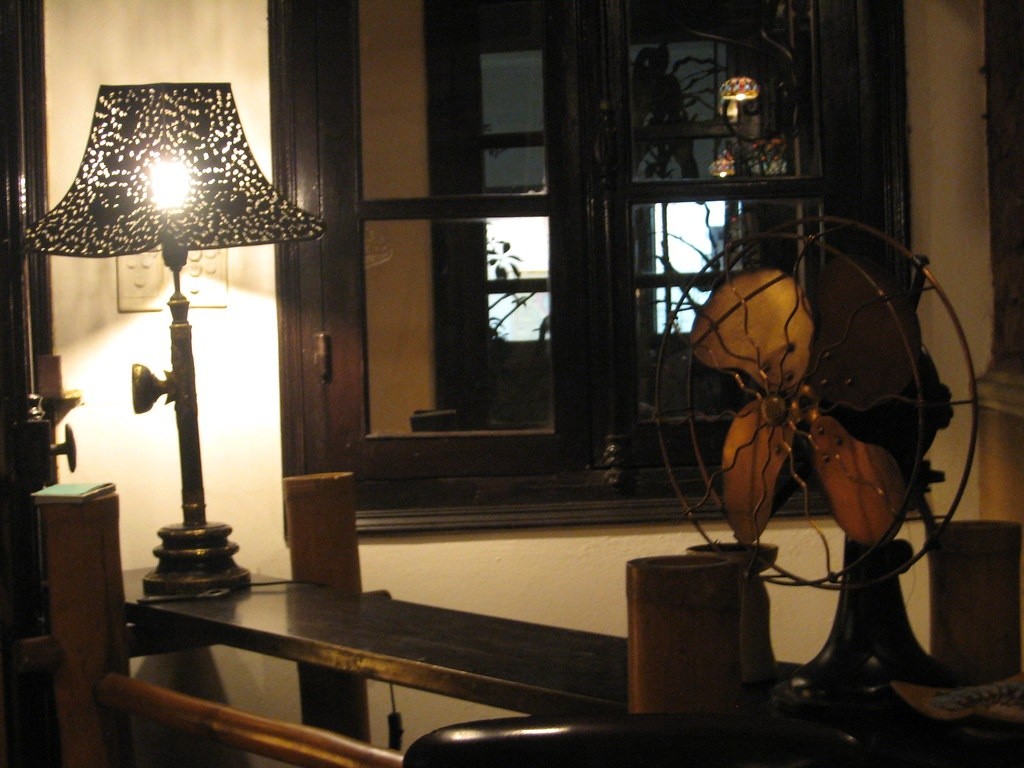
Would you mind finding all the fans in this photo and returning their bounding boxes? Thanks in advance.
[646,211,992,713]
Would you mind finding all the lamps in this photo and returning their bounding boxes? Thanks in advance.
[0,81,328,597]
